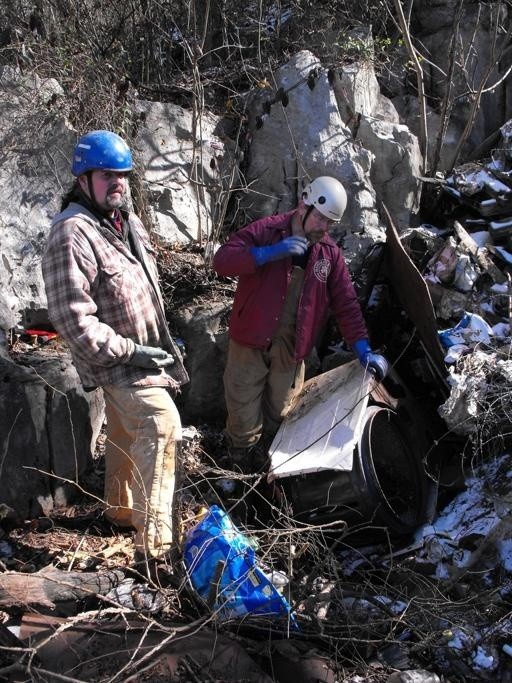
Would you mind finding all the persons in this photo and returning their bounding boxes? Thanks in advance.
[41,129,194,591]
[210,175,388,473]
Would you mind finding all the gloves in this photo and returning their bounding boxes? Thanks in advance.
[250,236,308,268]
[351,339,388,383]
[126,343,174,368]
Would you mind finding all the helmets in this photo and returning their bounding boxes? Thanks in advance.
[302,176,348,221]
[71,130,134,176]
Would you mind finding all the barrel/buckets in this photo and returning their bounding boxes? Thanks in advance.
[274,403,440,542]
